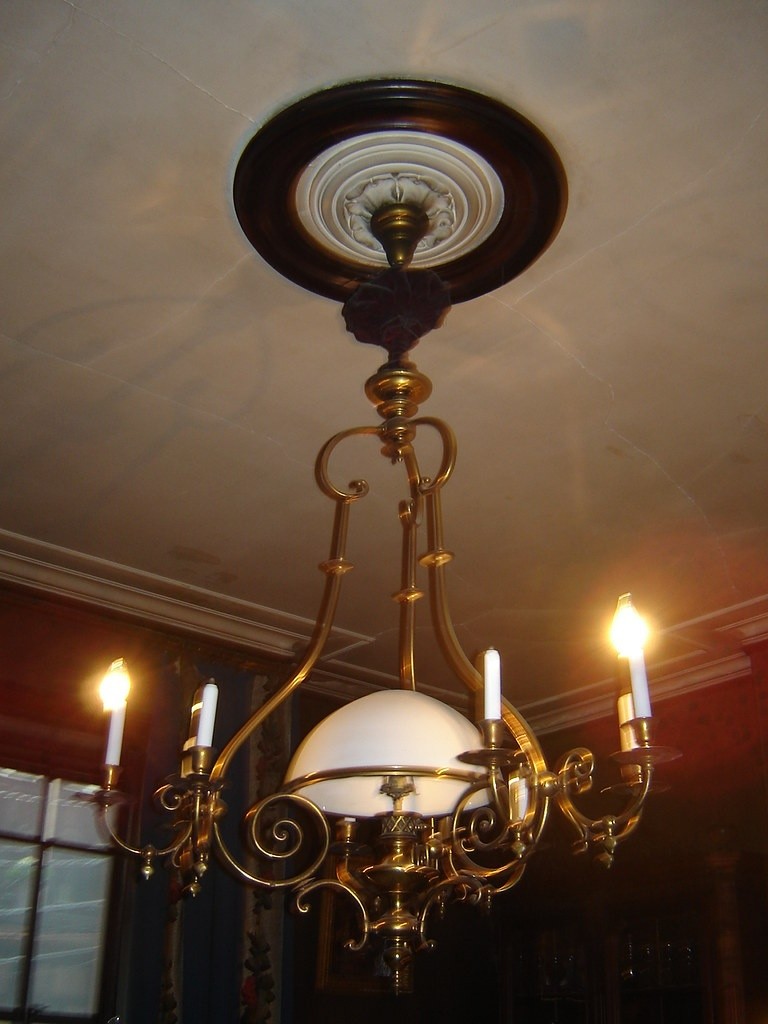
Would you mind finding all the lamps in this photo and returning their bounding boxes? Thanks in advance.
[74,77,658,1011]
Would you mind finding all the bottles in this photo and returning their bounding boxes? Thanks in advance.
[517,948,577,994]
[620,922,696,985]
